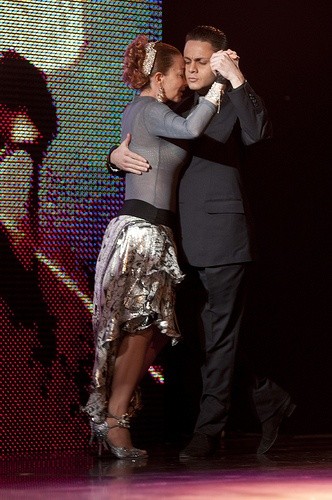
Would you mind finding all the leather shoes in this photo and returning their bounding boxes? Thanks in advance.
[256,399,296,453]
[179,451,214,459]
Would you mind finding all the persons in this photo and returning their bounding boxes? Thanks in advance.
[107,25,295,459]
[82,34,240,459]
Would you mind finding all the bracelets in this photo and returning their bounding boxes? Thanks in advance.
[214,72,227,84]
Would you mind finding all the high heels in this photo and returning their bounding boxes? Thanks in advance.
[89,412,150,459]
